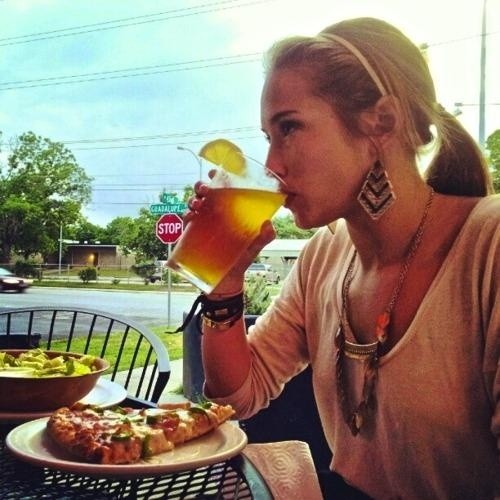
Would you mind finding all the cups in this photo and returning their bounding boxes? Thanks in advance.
[165,150,291,296]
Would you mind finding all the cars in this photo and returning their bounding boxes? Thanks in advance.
[0,266,37,294]
[244,263,281,285]
[150,271,163,282]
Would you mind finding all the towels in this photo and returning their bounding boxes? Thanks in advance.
[208,429,326,500]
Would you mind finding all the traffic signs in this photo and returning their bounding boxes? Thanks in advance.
[149,204,188,215]
[160,194,179,203]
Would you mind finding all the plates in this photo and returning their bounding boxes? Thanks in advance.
[5,409,249,479]
[0,381,132,424]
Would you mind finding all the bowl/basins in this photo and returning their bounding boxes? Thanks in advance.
[0,348,111,411]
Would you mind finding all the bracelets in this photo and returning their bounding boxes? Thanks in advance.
[168,290,246,336]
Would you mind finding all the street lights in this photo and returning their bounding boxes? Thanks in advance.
[41,218,63,276]
[176,146,204,183]
[454,100,500,149]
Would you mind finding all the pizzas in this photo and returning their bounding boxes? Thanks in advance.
[46,402,237,465]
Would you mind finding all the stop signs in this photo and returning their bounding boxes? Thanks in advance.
[155,214,183,244]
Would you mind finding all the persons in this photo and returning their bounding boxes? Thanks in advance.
[183,16,500,499]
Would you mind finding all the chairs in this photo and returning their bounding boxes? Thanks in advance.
[1,304,172,500]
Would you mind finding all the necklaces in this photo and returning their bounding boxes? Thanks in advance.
[331,179,434,436]
